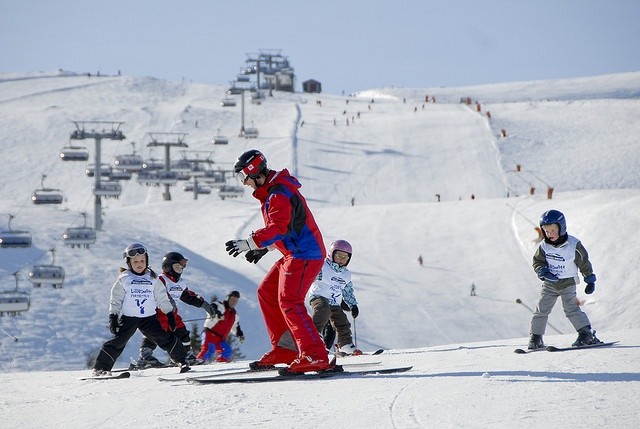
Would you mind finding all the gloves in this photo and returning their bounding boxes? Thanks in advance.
[225,241,249,257]
[171,298,176,311]
[239,336,244,343]
[585,283,594,294]
[215,310,223,318]
[245,247,268,264]
[352,305,358,318]
[201,301,215,315]
[538,268,559,282]
[109,313,119,334]
[166,311,176,331]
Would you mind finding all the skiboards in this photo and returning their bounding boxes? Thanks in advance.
[76,357,205,380]
[157,360,414,385]
[514,338,621,355]
[326,347,388,358]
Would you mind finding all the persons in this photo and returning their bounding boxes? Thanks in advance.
[309,239,362,356]
[95,244,187,374]
[322,300,349,354]
[435,194,440,202]
[224,150,331,373]
[527,210,599,348]
[137,252,218,369]
[417,254,423,265]
[195,291,245,364]
[472,194,475,199]
[351,196,355,206]
[469,282,476,296]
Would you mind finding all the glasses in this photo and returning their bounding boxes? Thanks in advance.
[334,253,349,260]
[123,248,147,258]
[236,155,264,185]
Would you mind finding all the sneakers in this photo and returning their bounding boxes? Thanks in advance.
[529,335,543,349]
[339,343,362,355]
[138,348,158,363]
[573,330,596,346]
[260,348,298,363]
[186,354,196,360]
[289,355,330,372]
[196,358,204,365]
[213,361,227,365]
[178,359,189,368]
[92,369,111,376]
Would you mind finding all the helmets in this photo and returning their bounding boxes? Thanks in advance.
[234,150,266,179]
[124,244,147,263]
[541,210,566,238]
[226,291,240,299]
[328,240,352,267]
[162,252,188,283]
